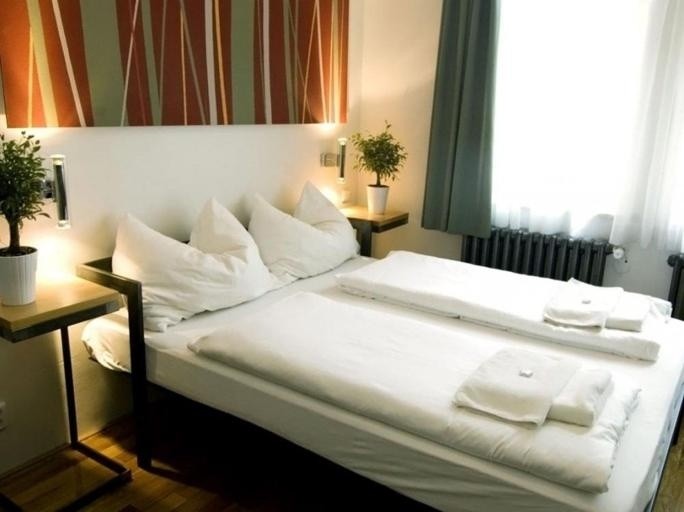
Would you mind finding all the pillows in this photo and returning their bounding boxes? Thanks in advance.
[248,181,359,282]
[113,199,279,332]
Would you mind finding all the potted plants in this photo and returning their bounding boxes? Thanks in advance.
[0,130,53,305]
[349,120,407,216]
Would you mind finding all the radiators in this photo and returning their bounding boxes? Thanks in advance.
[461,224,624,287]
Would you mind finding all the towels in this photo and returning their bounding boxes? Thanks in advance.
[544,273,624,331]
[452,344,574,430]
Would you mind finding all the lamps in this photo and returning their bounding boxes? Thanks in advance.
[320,137,346,184]
[43,155,71,231]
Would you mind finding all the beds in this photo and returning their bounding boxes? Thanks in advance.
[76,227,684,512]
